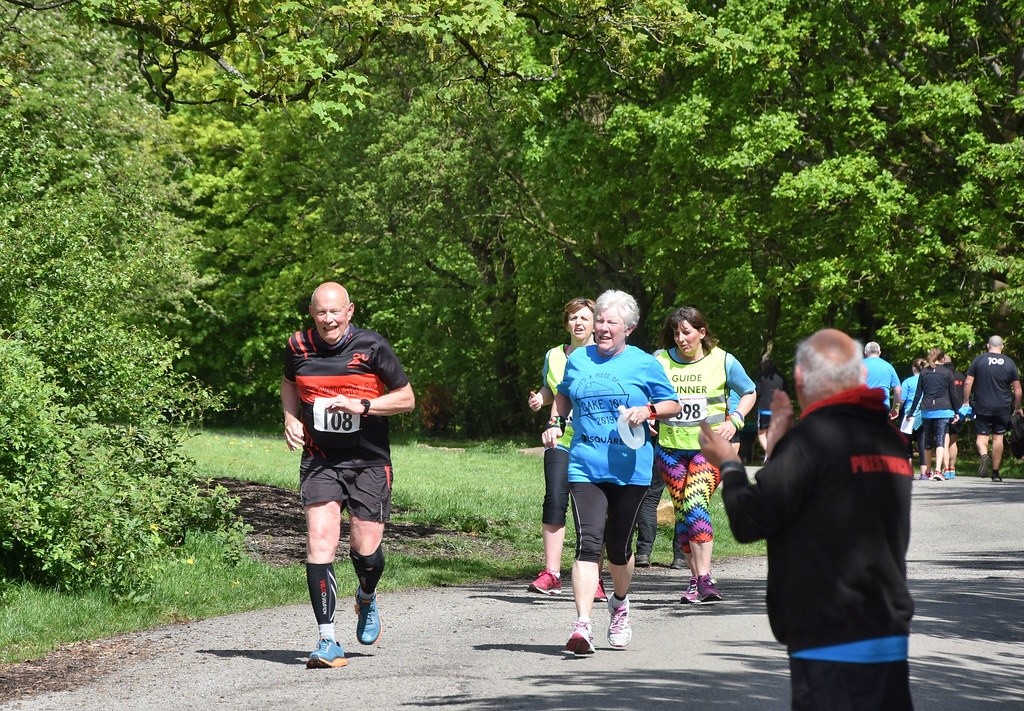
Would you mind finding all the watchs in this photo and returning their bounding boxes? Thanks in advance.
[361,398,371,417]
[646,403,658,420]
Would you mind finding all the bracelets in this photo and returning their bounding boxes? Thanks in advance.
[727,410,745,431]
[548,415,566,435]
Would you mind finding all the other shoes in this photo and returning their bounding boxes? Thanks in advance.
[634,552,650,567]
[671,557,690,570]
[924,469,945,481]
[942,469,955,480]
[979,454,990,479]
[992,473,1002,482]
[918,473,927,480]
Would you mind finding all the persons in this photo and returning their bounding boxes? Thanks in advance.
[634,349,689,569]
[862,341,967,482]
[542,288,682,655]
[729,358,783,465]
[281,282,416,670]
[646,306,760,606]
[963,335,1024,482]
[698,327,915,711]
[528,296,608,602]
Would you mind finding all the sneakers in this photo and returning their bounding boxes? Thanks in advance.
[697,570,722,602]
[355,584,382,646]
[307,637,348,669]
[607,592,632,646]
[565,621,596,655]
[527,568,562,595]
[593,578,609,602]
[680,577,701,604]
